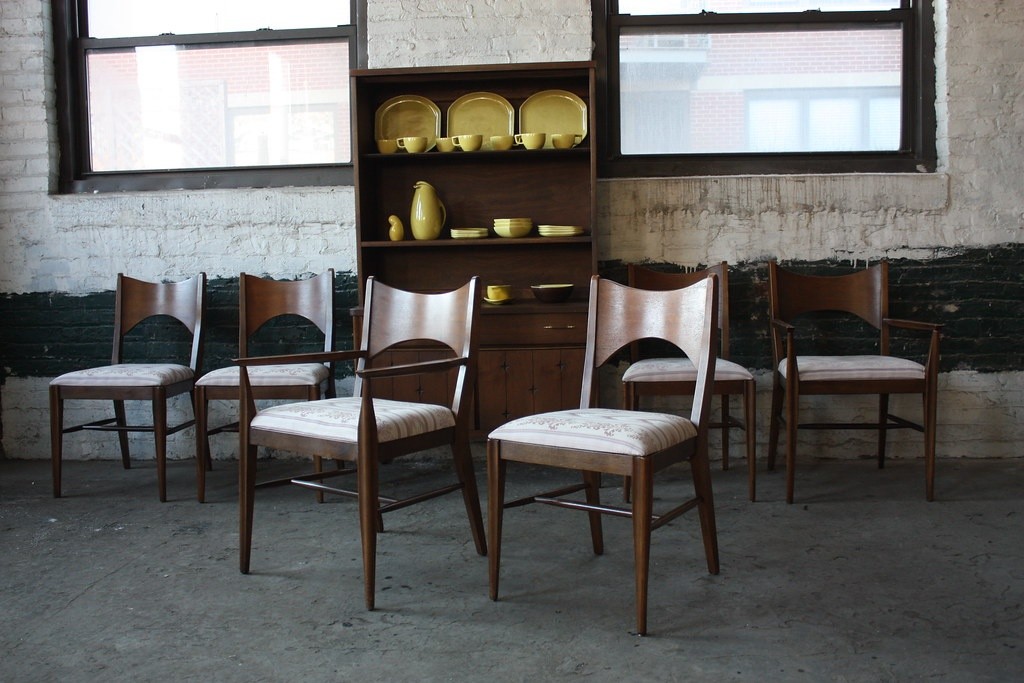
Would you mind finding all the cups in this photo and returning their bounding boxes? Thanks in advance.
[397,137,427,154]
[551,133,582,149]
[452,135,482,151]
[437,138,457,152]
[515,133,546,150]
[376,140,400,154]
[486,285,513,300]
[490,135,519,150]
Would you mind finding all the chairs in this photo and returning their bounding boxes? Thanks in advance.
[230,275,488,610]
[767,260,947,504]
[623,263,757,503]
[486,273,720,636]
[48,271,212,503]
[195,269,343,503]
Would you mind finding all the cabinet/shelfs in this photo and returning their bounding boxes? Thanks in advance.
[349,59,601,465]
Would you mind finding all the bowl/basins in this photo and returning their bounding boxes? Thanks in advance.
[530,284,574,304]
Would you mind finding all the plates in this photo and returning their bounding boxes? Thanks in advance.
[518,90,587,149]
[484,296,515,305]
[451,228,489,238]
[538,225,585,236]
[493,219,533,238]
[375,94,441,152]
[447,92,515,150]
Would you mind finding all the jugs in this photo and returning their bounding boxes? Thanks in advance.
[410,181,446,240]
